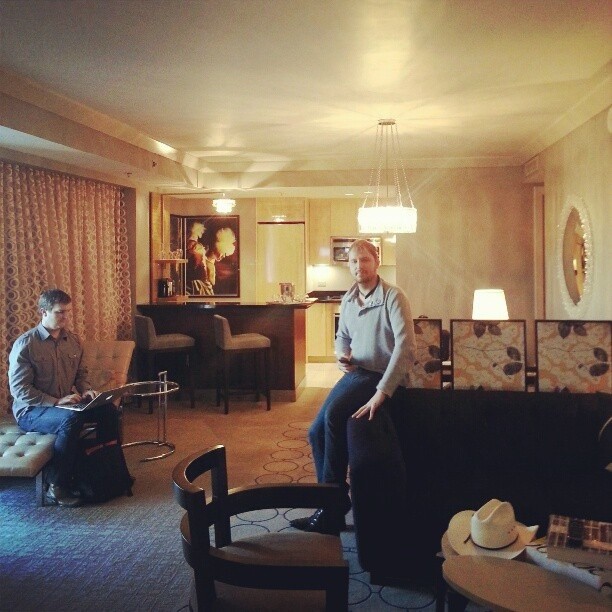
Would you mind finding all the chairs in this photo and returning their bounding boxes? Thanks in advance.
[213,314,272,414]
[134,315,197,415]
[172,444,349,612]
[0,341,136,507]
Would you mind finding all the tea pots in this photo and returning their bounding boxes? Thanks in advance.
[279,283,295,301]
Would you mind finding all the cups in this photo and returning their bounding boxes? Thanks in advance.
[582,521,601,551]
[548,515,568,549]
[596,521,612,552]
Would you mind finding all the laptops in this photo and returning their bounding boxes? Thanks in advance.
[54,383,135,412]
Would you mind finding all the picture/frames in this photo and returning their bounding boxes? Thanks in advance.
[184,216,241,298]
[170,214,185,295]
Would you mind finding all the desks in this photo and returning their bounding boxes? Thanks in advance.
[120,381,175,462]
[434,554,612,611]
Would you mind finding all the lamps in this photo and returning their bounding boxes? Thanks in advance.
[211,199,235,212]
[471,288,509,320]
[354,119,418,234]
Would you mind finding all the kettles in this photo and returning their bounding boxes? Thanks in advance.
[161,277,172,296]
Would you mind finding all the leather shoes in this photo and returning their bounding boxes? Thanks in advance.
[289,514,311,530]
[306,493,351,531]
[45,483,82,508]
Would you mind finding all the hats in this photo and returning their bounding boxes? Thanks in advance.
[446,498,540,560]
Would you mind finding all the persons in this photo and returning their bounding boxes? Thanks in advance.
[8,289,117,506]
[289,240,417,534]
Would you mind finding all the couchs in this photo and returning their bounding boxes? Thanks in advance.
[346,386,612,586]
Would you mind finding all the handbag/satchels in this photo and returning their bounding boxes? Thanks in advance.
[78,424,134,503]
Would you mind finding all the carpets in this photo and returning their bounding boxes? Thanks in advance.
[0,387,612,612]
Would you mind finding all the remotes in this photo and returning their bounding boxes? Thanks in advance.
[338,356,353,366]
